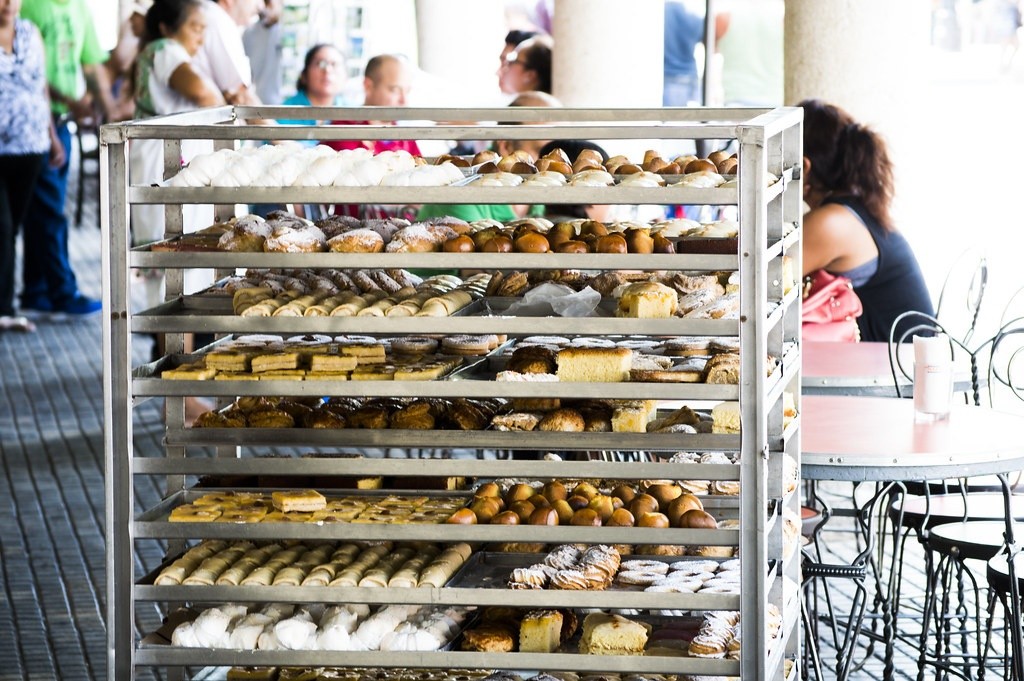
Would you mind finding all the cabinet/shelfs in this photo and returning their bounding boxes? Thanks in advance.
[99,102,805,681]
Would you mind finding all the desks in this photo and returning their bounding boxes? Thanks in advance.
[802,393,1024,681]
[800,340,995,397]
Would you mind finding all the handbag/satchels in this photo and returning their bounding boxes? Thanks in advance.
[800,270,863,344]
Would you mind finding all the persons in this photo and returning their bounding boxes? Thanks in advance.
[248,44,493,222]
[242,0,286,106]
[501,139,618,229]
[795,100,937,344]
[19,0,118,322]
[187,0,301,278]
[76,0,157,133]
[496,0,553,104]
[123,0,254,381]
[664,0,734,128]
[0,0,65,334]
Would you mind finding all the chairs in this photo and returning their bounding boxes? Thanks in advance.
[600,250,1024,681]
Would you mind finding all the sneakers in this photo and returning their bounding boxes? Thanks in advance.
[20,295,50,320]
[50,295,102,320]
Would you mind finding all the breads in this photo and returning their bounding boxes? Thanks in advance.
[157,142,794,681]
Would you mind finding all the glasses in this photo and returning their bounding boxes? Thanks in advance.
[503,59,525,67]
